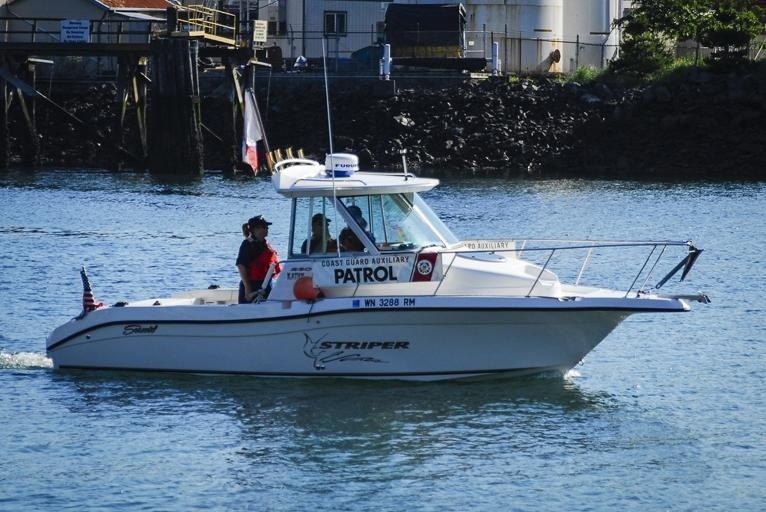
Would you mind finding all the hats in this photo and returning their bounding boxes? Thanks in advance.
[312,214,331,222]
[249,215,272,225]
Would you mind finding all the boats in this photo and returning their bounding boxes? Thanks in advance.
[42,33,715,389]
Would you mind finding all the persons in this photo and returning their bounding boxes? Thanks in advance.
[338,205,377,252]
[235,214,279,305]
[300,212,340,252]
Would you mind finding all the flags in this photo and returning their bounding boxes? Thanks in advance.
[240,88,264,177]
